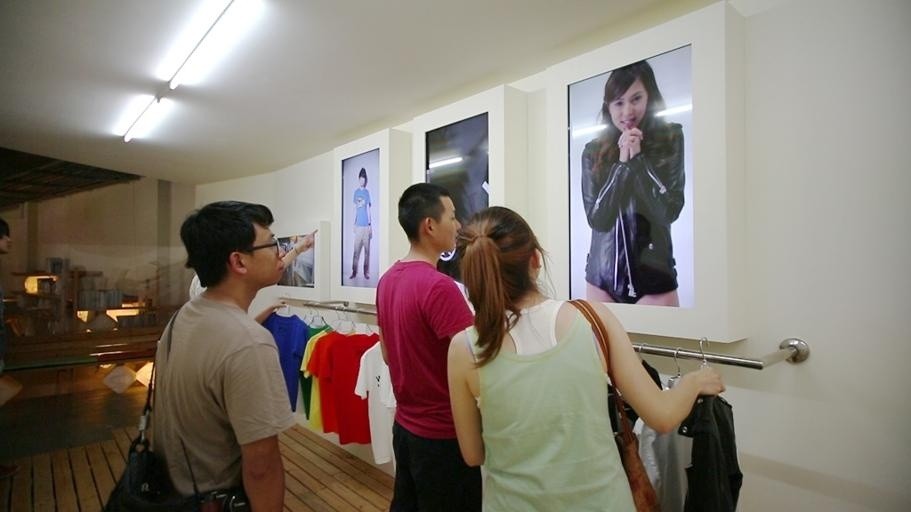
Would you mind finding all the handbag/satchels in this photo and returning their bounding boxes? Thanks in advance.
[616,431,659,512]
[103,436,201,512]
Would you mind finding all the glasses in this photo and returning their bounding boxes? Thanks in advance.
[244,238,280,254]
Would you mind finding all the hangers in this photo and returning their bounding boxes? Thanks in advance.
[277,296,379,336]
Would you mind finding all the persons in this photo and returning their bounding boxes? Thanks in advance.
[348,168,373,280]
[148,197,296,511]
[186,227,322,327]
[0,218,12,254]
[579,62,686,307]
[373,180,485,512]
[445,206,727,512]
